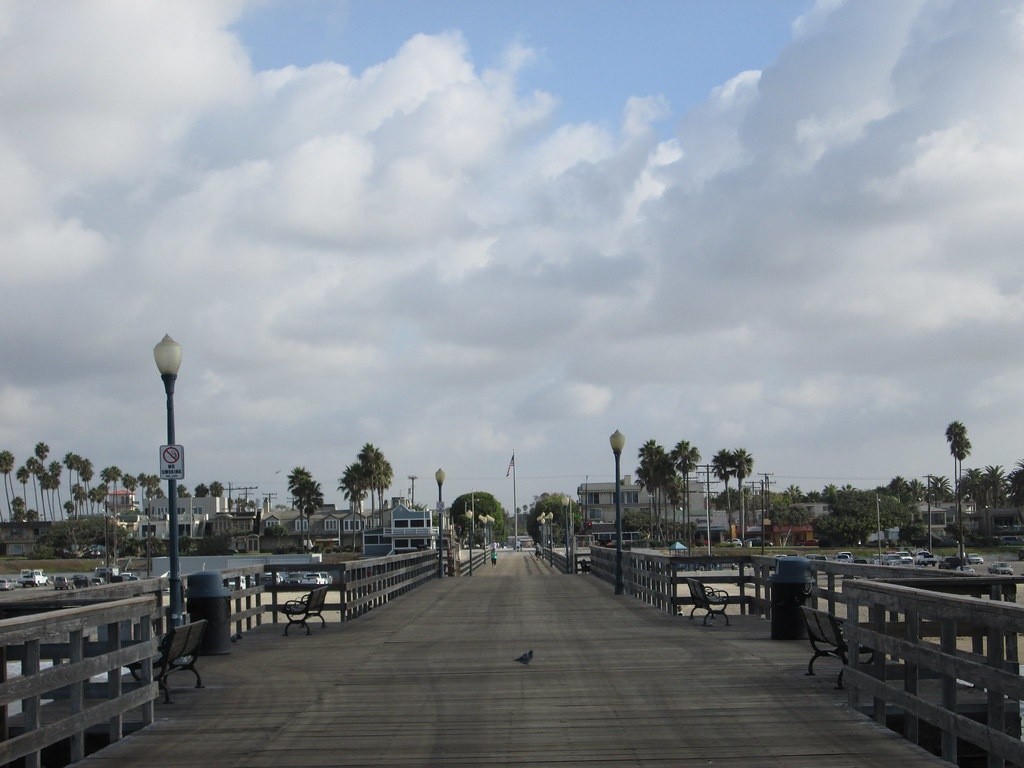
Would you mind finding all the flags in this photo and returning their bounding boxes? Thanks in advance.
[506,455,514,477]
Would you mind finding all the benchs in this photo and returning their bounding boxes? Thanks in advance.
[800,605,873,690]
[123,619,211,704]
[278,586,329,636]
[687,578,731,627]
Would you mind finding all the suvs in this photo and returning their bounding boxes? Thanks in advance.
[17,568,48,587]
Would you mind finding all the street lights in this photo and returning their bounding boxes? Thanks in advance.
[152,332,186,629]
[478,514,495,564]
[435,468,445,578]
[548,511,553,567]
[537,512,545,560]
[609,429,625,595]
[466,509,473,576]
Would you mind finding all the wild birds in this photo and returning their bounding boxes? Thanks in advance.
[513,649,533,664]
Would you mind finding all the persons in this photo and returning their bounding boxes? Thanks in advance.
[490,540,541,568]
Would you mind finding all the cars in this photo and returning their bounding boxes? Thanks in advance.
[228,570,335,591]
[53,576,74,590]
[0,578,15,590]
[773,548,939,567]
[72,566,138,590]
[939,557,966,571]
[987,561,1013,576]
[744,538,773,547]
[722,538,742,546]
[895,551,913,564]
[964,553,984,564]
